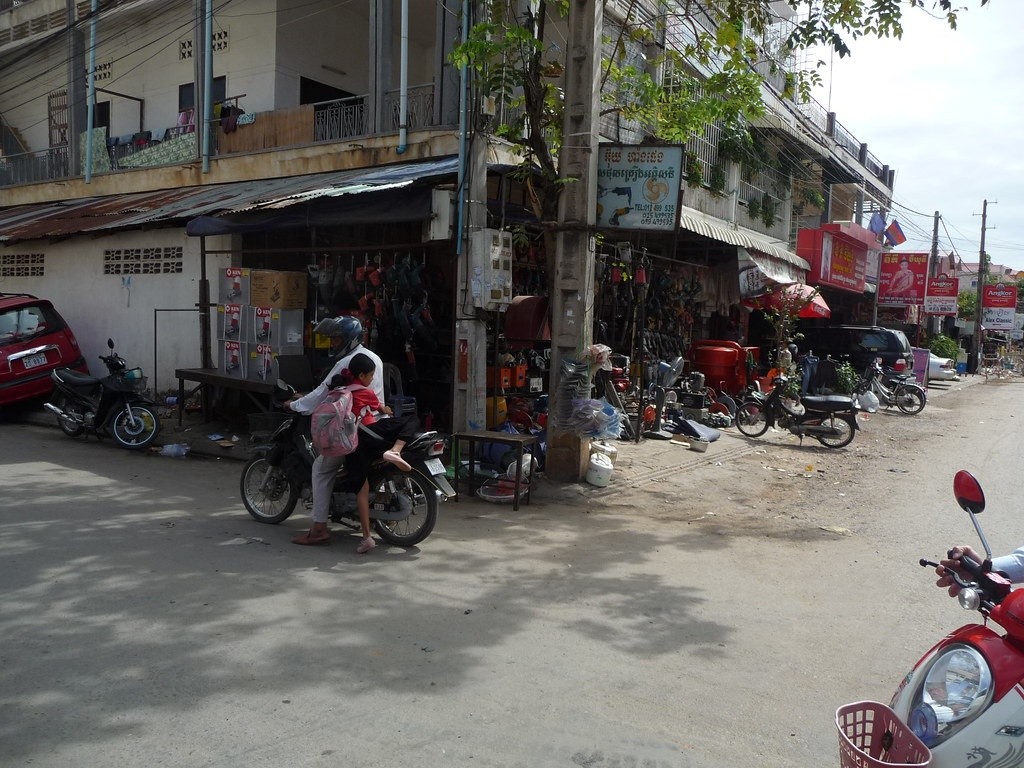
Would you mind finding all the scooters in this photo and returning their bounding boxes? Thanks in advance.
[833,469,1024,768]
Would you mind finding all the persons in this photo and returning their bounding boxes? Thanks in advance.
[936,545,1024,598]
[327,354,412,551]
[889,260,914,292]
[282,314,389,544]
[768,350,792,393]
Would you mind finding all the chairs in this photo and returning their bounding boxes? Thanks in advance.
[382,362,417,417]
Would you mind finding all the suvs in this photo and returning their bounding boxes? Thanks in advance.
[0,292,91,408]
[799,324,915,388]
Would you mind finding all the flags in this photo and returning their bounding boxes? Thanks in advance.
[884,220,907,246]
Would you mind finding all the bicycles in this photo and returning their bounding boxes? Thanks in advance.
[857,357,927,415]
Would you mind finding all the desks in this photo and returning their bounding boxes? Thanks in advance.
[453,430,539,513]
[174,368,278,428]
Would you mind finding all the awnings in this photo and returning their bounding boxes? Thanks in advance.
[0,157,460,240]
[748,114,829,160]
[680,207,811,270]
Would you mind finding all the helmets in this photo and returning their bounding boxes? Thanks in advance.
[313,315,362,366]
[122,368,143,385]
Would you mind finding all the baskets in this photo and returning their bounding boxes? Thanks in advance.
[834,700,931,768]
[119,376,148,402]
[862,367,880,382]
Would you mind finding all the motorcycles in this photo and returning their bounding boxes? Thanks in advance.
[734,366,862,449]
[42,337,163,452]
[239,378,458,548]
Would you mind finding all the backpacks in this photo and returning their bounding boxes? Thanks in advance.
[311,383,369,456]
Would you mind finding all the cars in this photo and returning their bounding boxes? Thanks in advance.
[910,346,956,380]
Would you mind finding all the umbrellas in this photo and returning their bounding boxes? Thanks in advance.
[773,284,830,319]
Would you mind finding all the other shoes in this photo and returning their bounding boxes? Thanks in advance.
[357,537,375,553]
[383,450,412,471]
[289,528,331,546]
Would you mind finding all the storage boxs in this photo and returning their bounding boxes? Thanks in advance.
[487,365,527,388]
[664,386,710,421]
[216,268,309,383]
[486,396,508,426]
